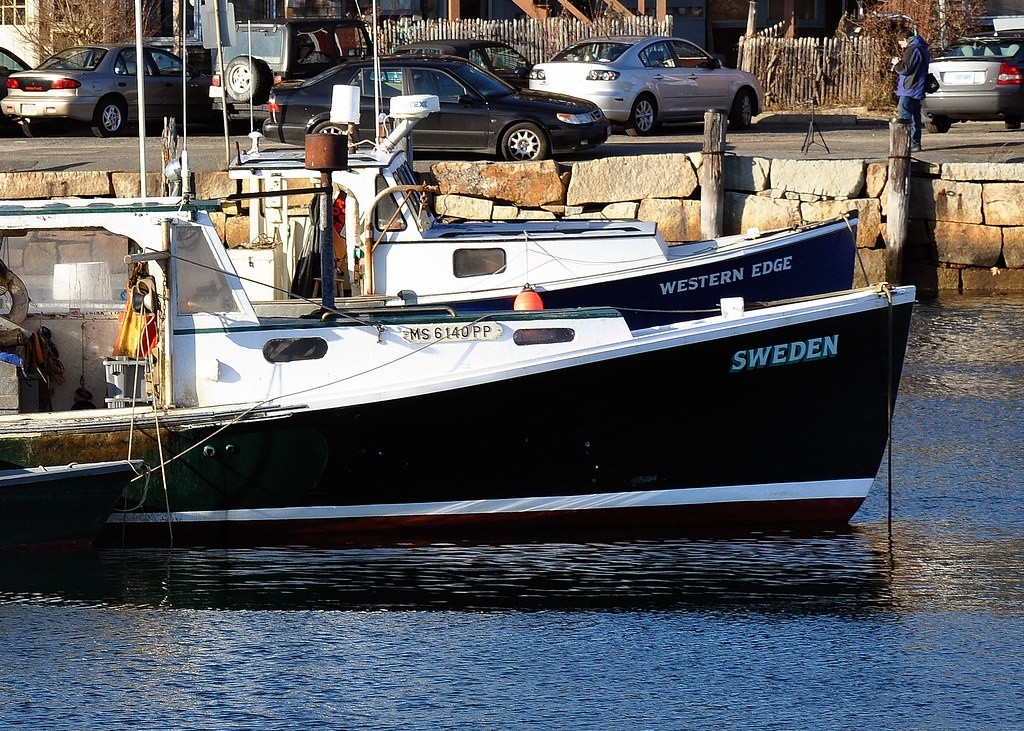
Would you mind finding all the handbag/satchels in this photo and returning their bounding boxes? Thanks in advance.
[925,73,939,93]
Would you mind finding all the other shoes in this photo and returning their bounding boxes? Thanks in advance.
[910,145,921,152]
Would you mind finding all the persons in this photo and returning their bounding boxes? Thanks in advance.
[892,29,930,151]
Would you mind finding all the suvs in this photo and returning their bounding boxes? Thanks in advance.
[209,18,384,120]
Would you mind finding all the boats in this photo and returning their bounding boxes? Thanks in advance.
[1,458,148,536]
[1,114,918,535]
[220,0,860,336]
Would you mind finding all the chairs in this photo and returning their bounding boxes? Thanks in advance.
[608,47,625,62]
[645,51,665,68]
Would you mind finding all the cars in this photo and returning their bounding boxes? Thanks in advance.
[0,48,56,130]
[1,43,214,139]
[528,35,766,137]
[367,39,537,94]
[996,44,1024,130]
[918,32,1024,133]
[262,54,613,161]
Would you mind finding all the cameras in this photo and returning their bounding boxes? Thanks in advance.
[891,58,903,72]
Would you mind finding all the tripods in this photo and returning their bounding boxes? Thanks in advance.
[801,97,831,156]
